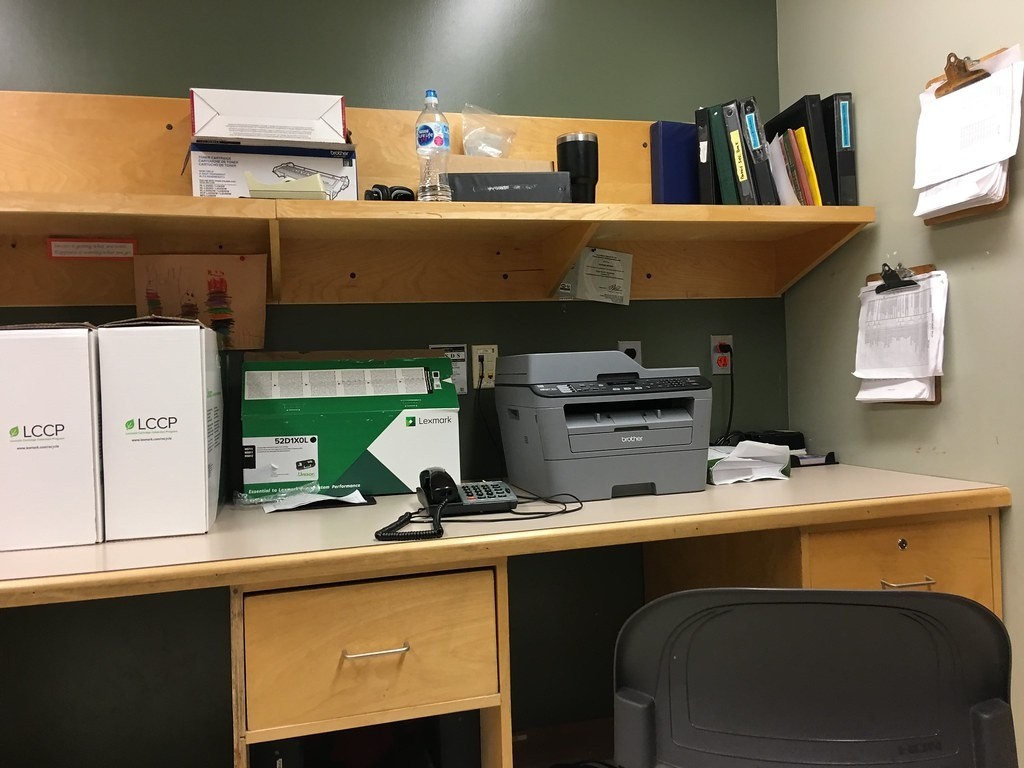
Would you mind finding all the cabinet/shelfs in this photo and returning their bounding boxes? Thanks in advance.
[2,87,1012,768]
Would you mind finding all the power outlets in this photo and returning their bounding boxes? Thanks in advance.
[471,345,499,390]
[616,340,643,366]
[711,335,734,375]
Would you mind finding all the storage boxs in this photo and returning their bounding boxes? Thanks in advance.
[179,86,360,200]
[3,312,224,555]
[240,349,460,507]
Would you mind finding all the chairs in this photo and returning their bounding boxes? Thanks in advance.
[610,586,1021,768]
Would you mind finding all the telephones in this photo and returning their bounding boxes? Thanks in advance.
[416,467,519,519]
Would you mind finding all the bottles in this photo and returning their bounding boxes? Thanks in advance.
[415,89,452,202]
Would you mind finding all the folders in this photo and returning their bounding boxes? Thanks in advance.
[649,92,859,206]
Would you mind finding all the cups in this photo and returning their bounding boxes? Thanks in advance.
[556,132,598,203]
